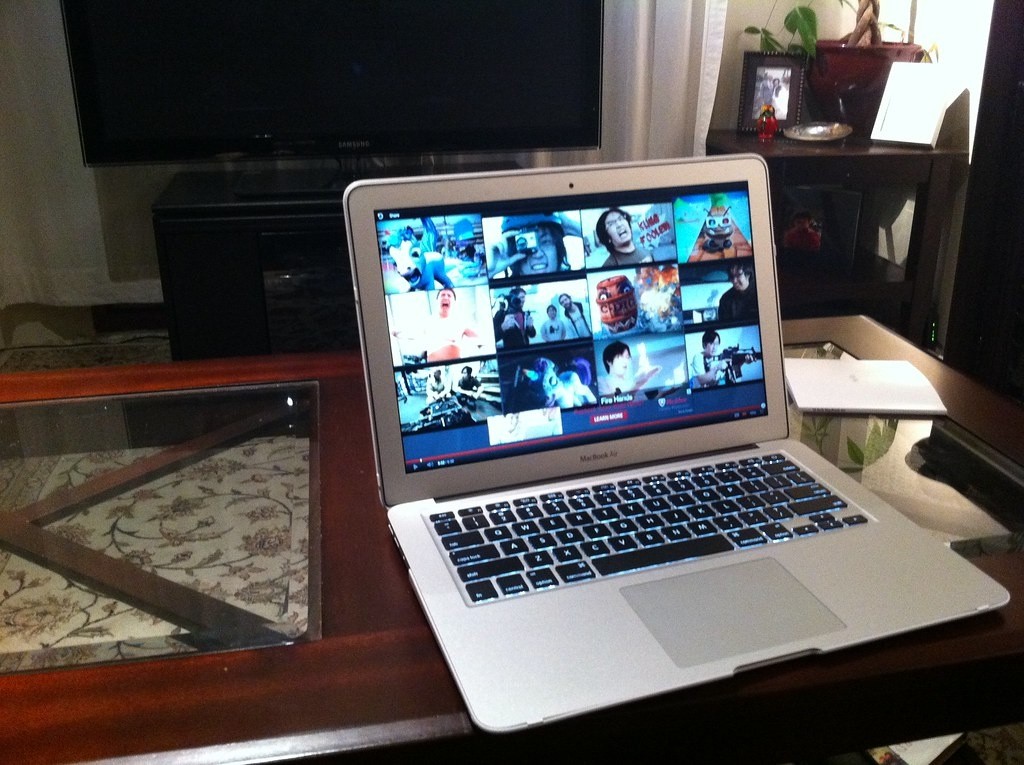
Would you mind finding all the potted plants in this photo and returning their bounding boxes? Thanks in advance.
[744,0,939,139]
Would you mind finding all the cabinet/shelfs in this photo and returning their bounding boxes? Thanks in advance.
[705,128,970,347]
[153,159,523,362]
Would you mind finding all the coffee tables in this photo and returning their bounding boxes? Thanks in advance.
[0,315,1024,765]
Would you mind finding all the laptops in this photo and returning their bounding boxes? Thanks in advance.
[344,151,1010,732]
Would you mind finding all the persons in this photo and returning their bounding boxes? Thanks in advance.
[390,209,761,408]
[760,78,784,115]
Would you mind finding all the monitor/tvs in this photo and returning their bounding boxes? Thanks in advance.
[59,0,604,195]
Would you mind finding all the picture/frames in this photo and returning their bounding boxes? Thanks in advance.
[871,62,951,150]
[738,51,807,138]
[773,186,863,279]
